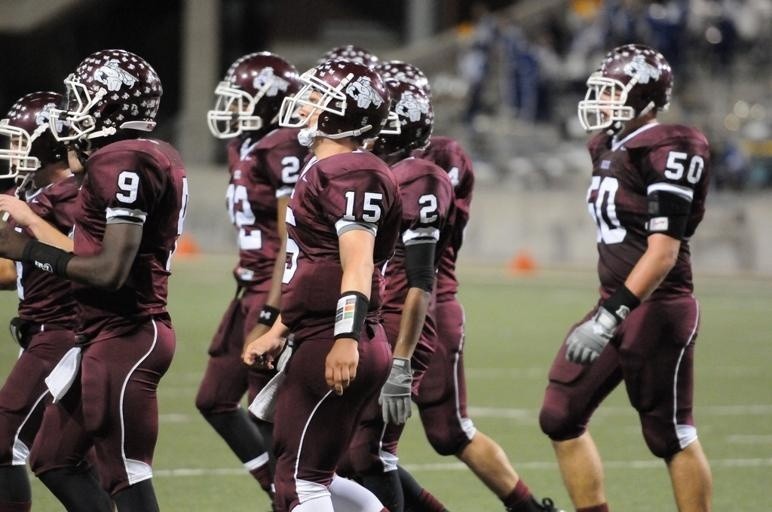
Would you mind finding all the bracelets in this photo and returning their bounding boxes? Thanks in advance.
[332,288,373,344]
[254,301,282,329]
[18,236,78,284]
[600,281,642,330]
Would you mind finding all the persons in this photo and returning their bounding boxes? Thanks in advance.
[192,50,314,512]
[374,58,561,511]
[268,43,406,512]
[537,40,715,510]
[430,1,771,201]
[380,76,454,509]
[1,49,190,511]
[0,89,117,512]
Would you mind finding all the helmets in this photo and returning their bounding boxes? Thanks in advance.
[49,49,163,141]
[0,92,66,191]
[576,44,674,130]
[207,52,301,140]
[316,44,377,69]
[374,61,429,89]
[370,77,434,157]
[279,58,391,146]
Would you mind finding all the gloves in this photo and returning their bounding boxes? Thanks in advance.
[377,356,413,425]
[563,305,619,365]
